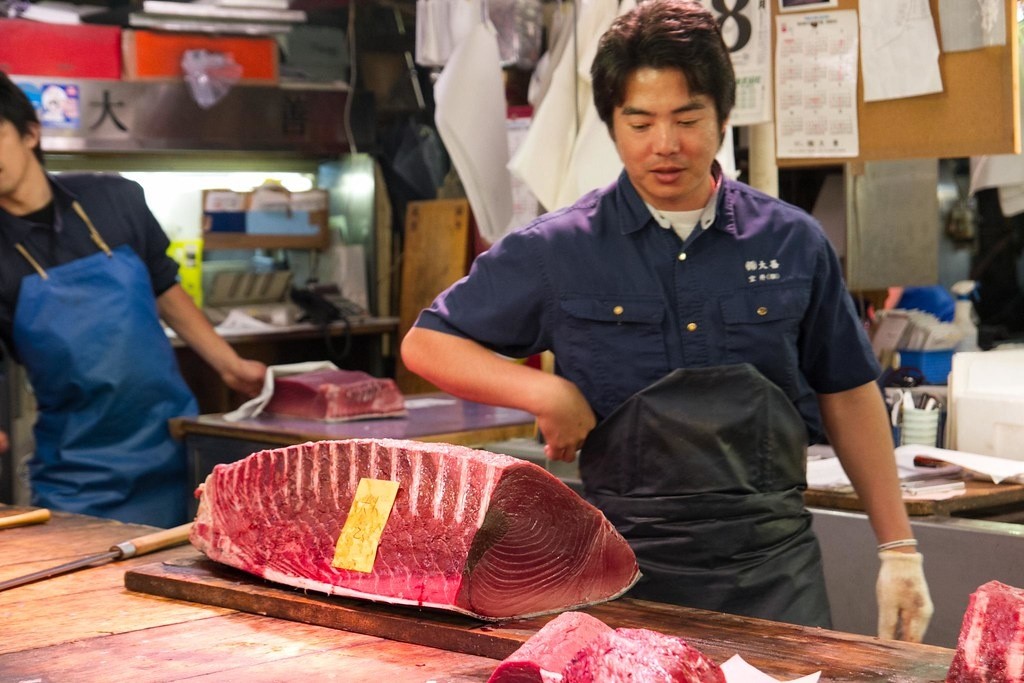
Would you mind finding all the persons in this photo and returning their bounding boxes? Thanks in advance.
[400,6,934,644]
[0,69,269,533]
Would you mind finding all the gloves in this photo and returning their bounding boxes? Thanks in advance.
[875,551,934,644]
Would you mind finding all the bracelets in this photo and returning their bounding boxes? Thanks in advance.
[877,538,923,552]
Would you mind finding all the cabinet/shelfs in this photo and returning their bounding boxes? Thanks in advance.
[6,75,402,408]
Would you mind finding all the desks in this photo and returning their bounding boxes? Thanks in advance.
[168,390,538,524]
[0,502,500,683]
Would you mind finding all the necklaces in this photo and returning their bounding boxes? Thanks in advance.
[708,173,716,202]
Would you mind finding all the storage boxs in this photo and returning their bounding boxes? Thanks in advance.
[395,196,470,392]
[122,29,281,87]
[0,16,122,81]
